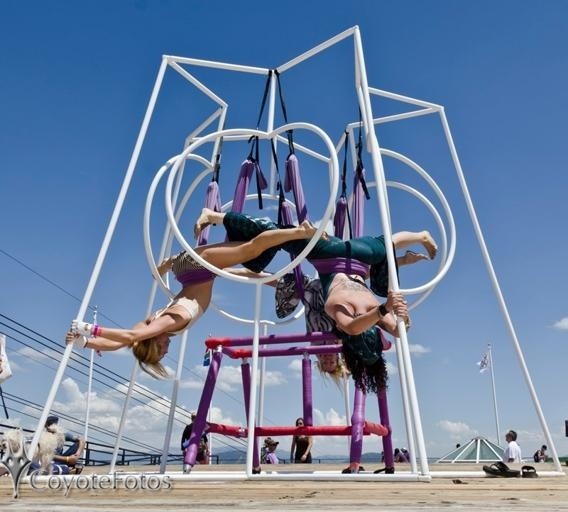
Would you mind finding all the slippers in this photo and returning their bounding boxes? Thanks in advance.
[483,463,538,478]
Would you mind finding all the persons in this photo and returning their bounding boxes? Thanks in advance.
[260,437,273,464]
[289,417,313,462]
[28,415,85,475]
[179,411,211,464]
[194,207,441,390]
[533,444,549,462]
[65,219,330,380]
[503,430,521,463]
[393,447,408,462]
[381,447,385,463]
[265,439,281,464]
[218,250,431,385]
[400,448,410,462]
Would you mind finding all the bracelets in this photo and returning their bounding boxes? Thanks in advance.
[83,335,89,349]
[376,301,391,320]
[304,453,308,456]
[92,324,102,339]
[405,315,413,331]
[66,455,69,463]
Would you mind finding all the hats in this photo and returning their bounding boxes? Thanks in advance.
[265,437,280,446]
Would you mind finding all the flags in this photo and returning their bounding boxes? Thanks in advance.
[476,351,489,374]
